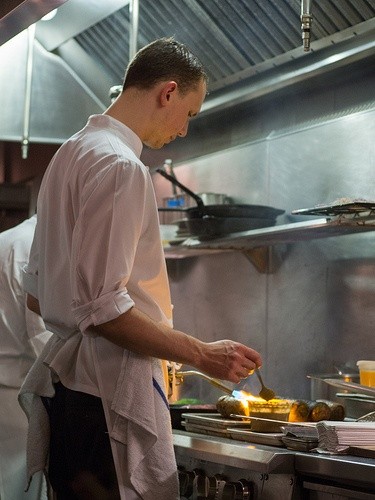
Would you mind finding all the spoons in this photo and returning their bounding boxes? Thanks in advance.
[254,364,275,401]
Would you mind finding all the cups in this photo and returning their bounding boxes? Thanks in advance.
[356,360,375,390]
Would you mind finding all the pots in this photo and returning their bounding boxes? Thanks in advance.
[154,169,285,241]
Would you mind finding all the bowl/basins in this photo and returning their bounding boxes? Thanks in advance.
[249,400,291,433]
[282,435,318,452]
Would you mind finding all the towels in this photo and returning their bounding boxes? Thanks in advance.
[110,348,180,500]
